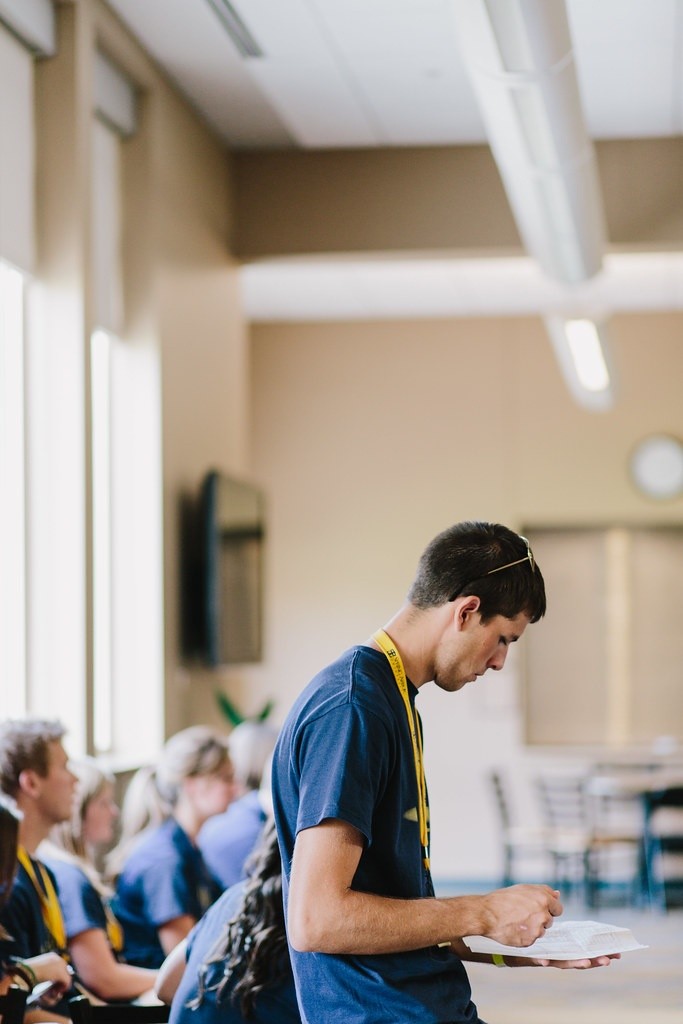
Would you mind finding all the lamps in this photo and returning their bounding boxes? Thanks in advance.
[448,1,610,285]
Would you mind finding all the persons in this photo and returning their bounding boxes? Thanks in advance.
[269,521,624,1024]
[0,718,287,1024]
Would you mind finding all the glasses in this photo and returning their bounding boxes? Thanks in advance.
[448,534,533,603]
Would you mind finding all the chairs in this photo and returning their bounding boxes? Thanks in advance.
[487,763,683,915]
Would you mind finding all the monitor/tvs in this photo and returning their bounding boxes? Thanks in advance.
[192,468,264,668]
